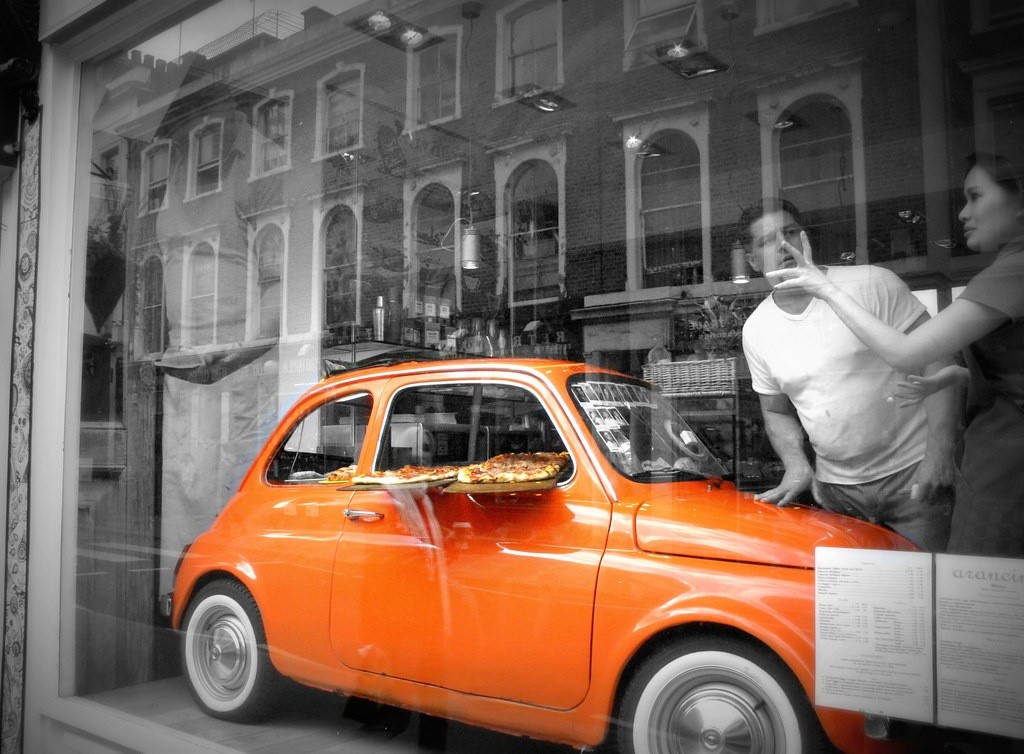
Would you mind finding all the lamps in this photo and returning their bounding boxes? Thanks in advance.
[775,109,794,130]
[720,3,751,286]
[461,1,483,271]
[641,145,661,157]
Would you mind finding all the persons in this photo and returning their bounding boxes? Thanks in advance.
[766,152,1024,558]
[739,197,952,553]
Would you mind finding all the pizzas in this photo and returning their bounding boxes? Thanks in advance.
[327,452,570,483]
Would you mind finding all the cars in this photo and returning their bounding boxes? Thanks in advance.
[159,358,926,754]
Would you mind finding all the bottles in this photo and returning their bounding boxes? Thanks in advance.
[372,295,389,342]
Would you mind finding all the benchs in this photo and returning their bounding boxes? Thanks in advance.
[274,448,355,482]
